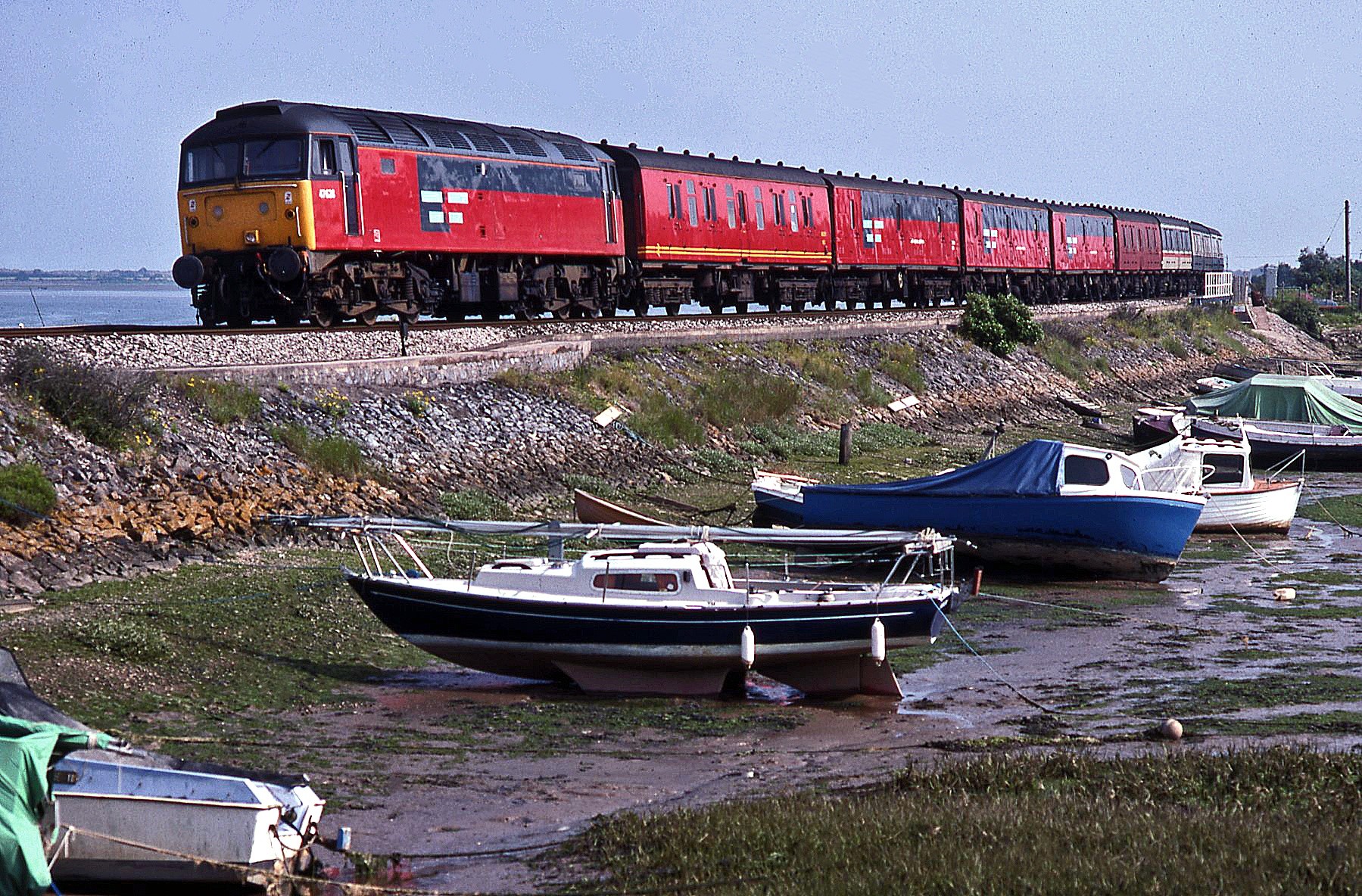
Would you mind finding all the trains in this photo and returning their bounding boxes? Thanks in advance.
[173,99,1226,325]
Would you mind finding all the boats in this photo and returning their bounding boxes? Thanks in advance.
[754,438,1217,585]
[1133,355,1362,468]
[45,757,330,883]
[1122,435,1306,534]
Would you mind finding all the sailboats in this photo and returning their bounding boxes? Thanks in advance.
[250,510,967,699]
